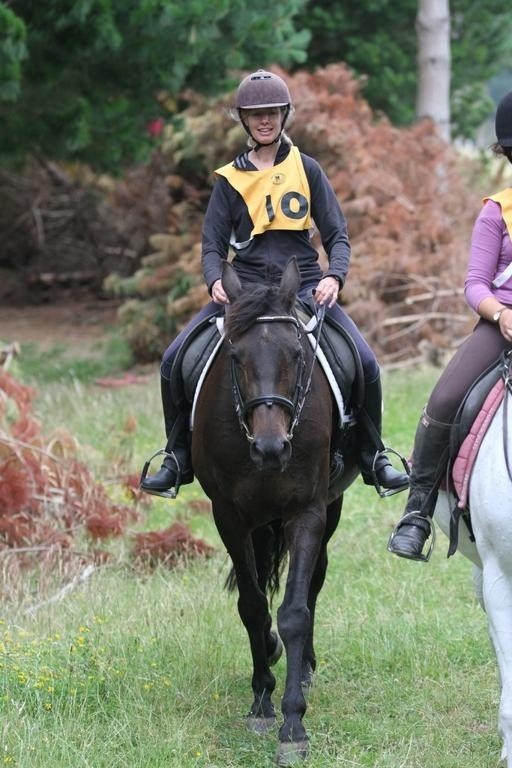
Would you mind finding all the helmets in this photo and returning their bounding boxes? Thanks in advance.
[236,69,290,109]
[496,91,512,148]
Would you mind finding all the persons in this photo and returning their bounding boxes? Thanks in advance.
[138,64,413,495]
[389,86,512,560]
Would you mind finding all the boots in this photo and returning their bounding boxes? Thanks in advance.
[361,376,410,488]
[141,369,193,489]
[390,410,458,556]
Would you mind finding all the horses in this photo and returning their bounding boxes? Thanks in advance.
[433,356,512,768]
[189,252,360,767]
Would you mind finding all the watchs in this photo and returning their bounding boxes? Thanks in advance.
[492,306,510,329]
